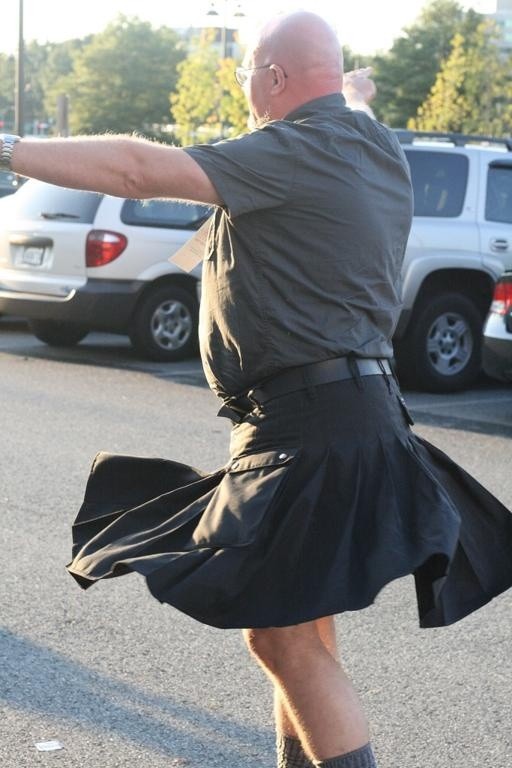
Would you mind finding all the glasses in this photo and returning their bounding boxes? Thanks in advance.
[234,65,288,87]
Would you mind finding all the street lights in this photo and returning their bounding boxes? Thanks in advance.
[205,0,248,60]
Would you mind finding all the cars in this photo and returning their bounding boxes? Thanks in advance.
[482,265,512,391]
[0,166,28,195]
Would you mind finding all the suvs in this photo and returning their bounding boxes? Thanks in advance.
[387,128,511,392]
[0,174,211,365]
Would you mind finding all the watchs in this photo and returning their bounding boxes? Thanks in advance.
[0,133,22,172]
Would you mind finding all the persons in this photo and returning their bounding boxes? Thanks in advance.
[0,9,511,768]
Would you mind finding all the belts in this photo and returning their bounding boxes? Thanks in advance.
[216,356,393,424]
